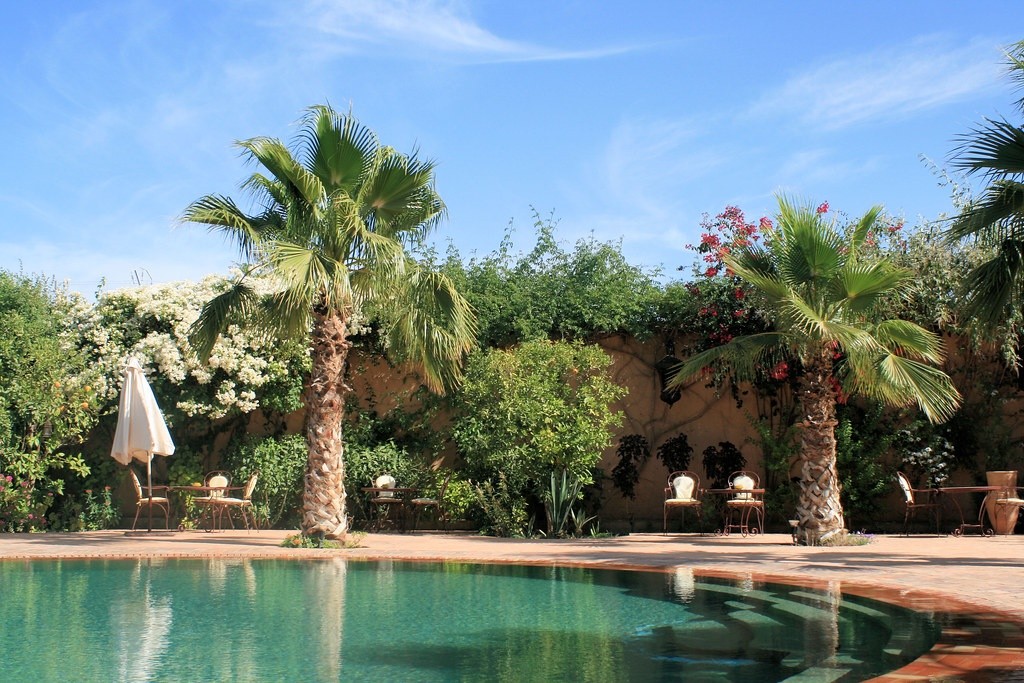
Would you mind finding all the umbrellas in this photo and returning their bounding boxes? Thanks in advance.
[110,356,177,536]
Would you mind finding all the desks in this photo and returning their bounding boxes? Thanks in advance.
[361,487,417,534]
[170,486,244,533]
[705,489,765,538]
[939,486,1004,538]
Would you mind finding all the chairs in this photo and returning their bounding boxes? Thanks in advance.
[129,465,170,532]
[370,471,402,533]
[662,471,704,537]
[409,475,451,535]
[991,486,1024,538]
[724,471,764,535]
[203,470,235,530]
[896,470,948,538]
[212,470,259,534]
